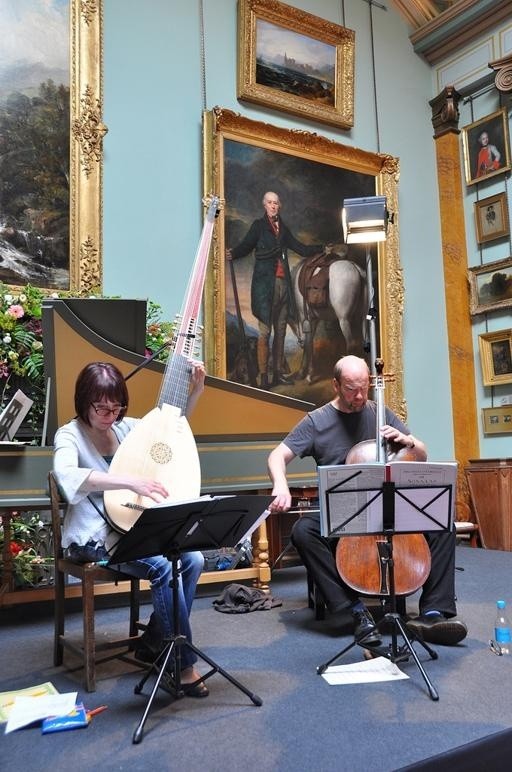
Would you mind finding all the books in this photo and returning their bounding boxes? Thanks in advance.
[316,460,457,538]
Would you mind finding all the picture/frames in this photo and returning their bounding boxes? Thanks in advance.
[477,327,512,387]
[472,191,511,245]
[234,0,358,131]
[480,405,512,435]
[0,1,109,305]
[198,103,409,436]
[462,108,512,188]
[463,256,512,318]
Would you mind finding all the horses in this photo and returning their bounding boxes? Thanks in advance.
[290,245,374,382]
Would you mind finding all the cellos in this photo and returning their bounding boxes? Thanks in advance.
[336,359,431,599]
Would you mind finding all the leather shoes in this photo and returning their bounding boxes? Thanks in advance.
[134,644,162,663]
[355,611,381,644]
[405,614,467,645]
[179,678,209,697]
[260,374,268,389]
[272,374,294,385]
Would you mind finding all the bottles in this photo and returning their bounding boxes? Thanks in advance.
[493,600,511,656]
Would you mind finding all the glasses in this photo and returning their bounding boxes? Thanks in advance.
[489,640,502,656]
[91,401,127,416]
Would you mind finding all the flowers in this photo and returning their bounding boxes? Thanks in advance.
[0,279,41,438]
[147,303,174,361]
[0,512,55,590]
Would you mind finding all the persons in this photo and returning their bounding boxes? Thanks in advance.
[52,357,212,697]
[496,345,506,362]
[226,190,332,388]
[267,354,469,648]
[485,206,497,232]
[474,131,502,179]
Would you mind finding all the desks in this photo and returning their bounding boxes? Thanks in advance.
[3,491,271,607]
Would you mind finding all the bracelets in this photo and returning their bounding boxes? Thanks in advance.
[406,434,415,448]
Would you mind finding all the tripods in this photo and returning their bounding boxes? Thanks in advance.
[317,538,441,702]
[132,560,263,745]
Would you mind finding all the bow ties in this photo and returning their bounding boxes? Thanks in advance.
[272,216,278,222]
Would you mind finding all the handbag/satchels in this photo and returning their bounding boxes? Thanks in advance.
[306,265,329,308]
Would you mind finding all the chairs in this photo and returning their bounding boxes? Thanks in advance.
[44,463,153,697]
[299,509,408,624]
